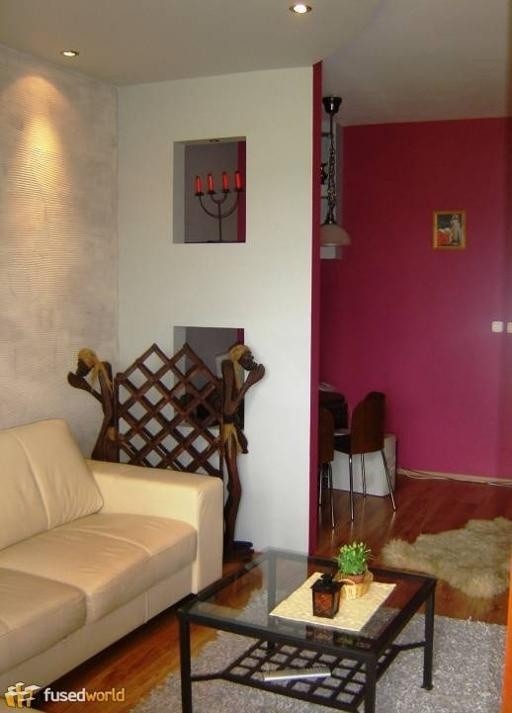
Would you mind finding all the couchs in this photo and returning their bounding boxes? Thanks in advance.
[0,418,228,712]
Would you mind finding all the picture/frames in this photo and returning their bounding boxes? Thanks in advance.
[432,209,468,250]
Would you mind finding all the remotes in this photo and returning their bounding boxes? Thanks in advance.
[263,668,331,682]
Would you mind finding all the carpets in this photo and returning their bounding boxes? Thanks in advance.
[128,585,507,712]
[380,515,512,599]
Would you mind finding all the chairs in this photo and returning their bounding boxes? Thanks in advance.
[318,406,335,528]
[327,391,397,521]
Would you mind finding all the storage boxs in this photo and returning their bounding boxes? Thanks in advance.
[328,433,395,497]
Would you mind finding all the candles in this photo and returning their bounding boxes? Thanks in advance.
[195,176,201,193]
[208,175,213,191]
[222,174,228,190]
[234,172,241,189]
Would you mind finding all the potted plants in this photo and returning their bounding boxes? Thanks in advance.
[334,542,373,598]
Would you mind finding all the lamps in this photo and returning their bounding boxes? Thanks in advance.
[319,95,351,247]
